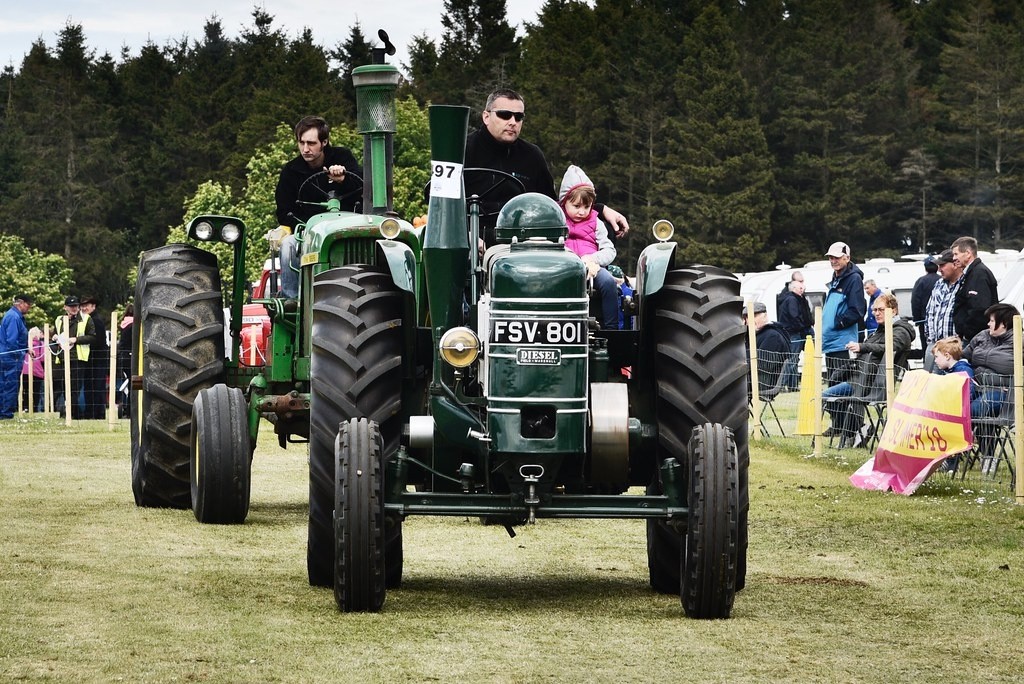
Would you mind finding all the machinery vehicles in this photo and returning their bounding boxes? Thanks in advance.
[305,103,748,618]
[121,64,423,523]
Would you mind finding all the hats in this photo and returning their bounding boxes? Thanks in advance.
[65,296,80,306]
[14,293,31,303]
[824,242,851,258]
[81,296,98,304]
[742,302,766,315]
[930,249,956,265]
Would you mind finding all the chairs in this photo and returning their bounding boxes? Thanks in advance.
[749,350,1015,490]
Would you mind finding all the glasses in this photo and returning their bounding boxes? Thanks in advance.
[487,110,526,122]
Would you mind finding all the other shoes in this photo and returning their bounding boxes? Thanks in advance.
[853,424,874,448]
[836,437,855,448]
[981,457,998,477]
[283,300,297,312]
[947,468,961,480]
[822,427,841,437]
[935,461,949,472]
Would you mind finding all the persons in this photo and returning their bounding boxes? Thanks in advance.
[924,249,965,372]
[822,242,867,437]
[463,89,629,258]
[911,255,942,359]
[742,301,792,398]
[0,293,31,420]
[22,326,60,413]
[52,296,96,420]
[608,265,634,330]
[931,336,975,471]
[778,281,812,393]
[822,293,916,449]
[950,236,999,350]
[79,296,106,420]
[555,165,620,330]
[120,305,133,419]
[776,271,815,388]
[274,115,363,313]
[961,303,1024,473]
[863,279,885,334]
